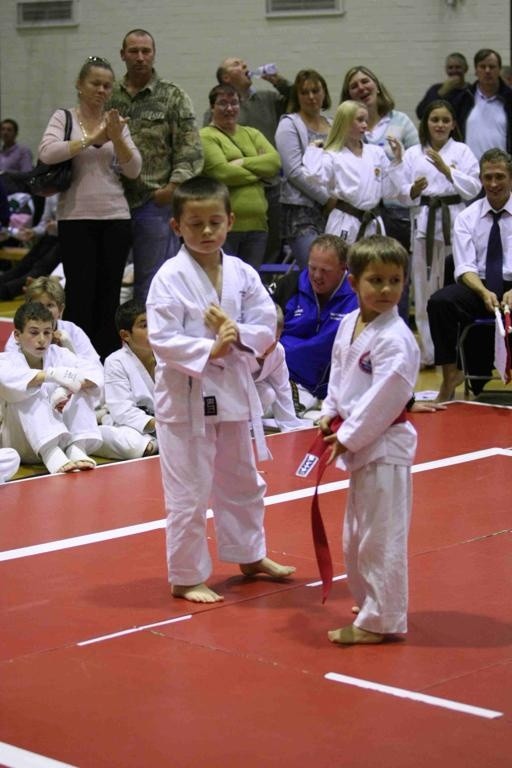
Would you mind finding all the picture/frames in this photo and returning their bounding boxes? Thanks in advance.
[14,0,79,30]
[263,0,347,22]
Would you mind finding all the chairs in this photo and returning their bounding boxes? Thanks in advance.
[455,316,512,404]
[261,246,312,287]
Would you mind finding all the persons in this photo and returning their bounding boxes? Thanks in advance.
[143,176,297,606]
[0,29,511,485]
[317,233,422,646]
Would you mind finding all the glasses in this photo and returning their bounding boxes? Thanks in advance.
[84,56,112,67]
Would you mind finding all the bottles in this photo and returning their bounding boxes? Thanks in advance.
[248,64,278,79]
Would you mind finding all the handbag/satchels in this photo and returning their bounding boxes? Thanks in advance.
[30,157,73,197]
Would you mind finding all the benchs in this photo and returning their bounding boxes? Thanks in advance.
[1,246,32,271]
